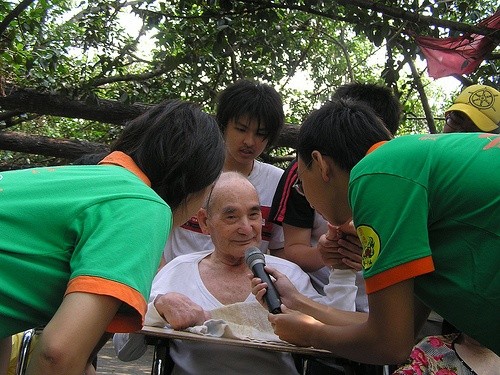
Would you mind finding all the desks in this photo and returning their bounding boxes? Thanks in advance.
[140,326,332,375]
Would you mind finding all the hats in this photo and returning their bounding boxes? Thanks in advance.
[445,84,500,131]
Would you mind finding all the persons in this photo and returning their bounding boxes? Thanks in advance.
[112,169,358,375]
[157,77,284,269]
[268,82,401,375]
[247,97,500,364]
[414,85,500,343]
[0,101,226,375]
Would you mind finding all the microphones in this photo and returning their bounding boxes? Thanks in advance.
[243,245,282,314]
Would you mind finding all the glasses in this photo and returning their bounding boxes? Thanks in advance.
[445,112,473,132]
[292,152,332,196]
